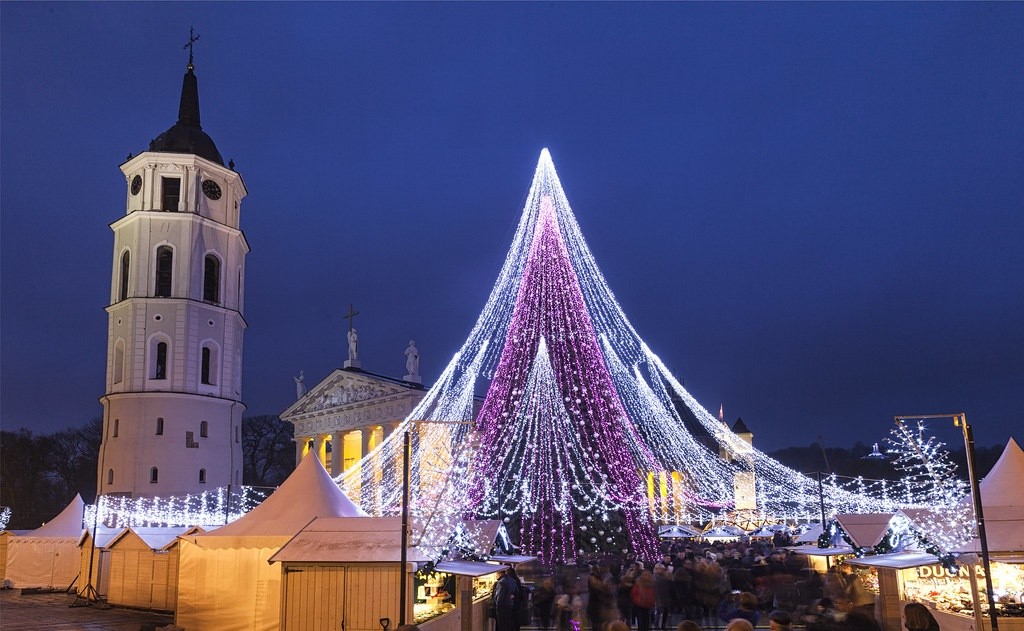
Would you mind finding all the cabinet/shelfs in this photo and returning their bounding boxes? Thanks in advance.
[899,601,1024,631]
[414,589,492,631]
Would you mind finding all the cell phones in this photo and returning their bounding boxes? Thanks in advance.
[729,588,742,595]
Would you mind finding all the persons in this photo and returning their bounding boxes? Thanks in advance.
[347,328,359,360]
[403,339,420,376]
[293,370,306,400]
[489,535,940,630]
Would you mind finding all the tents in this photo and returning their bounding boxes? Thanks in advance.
[955,437,1024,507]
[3,492,107,591]
[174,445,371,631]
